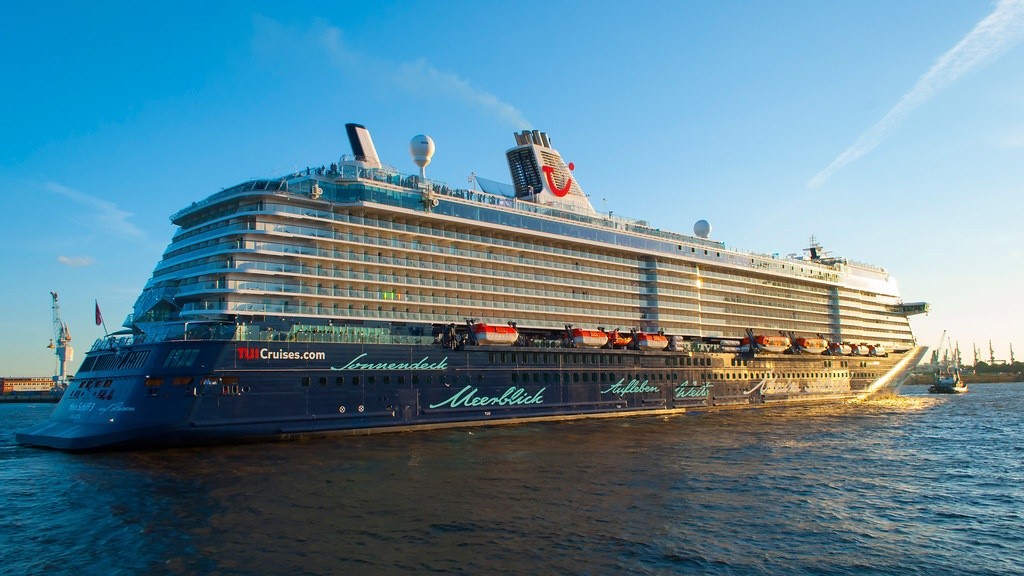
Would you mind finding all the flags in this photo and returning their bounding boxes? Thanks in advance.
[94,301,103,326]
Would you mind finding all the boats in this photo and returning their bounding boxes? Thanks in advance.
[927,366,967,393]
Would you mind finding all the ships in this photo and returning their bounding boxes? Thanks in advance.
[15,122,930,453]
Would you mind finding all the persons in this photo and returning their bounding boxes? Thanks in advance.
[179,322,239,339]
[515,333,576,348]
[257,326,384,344]
